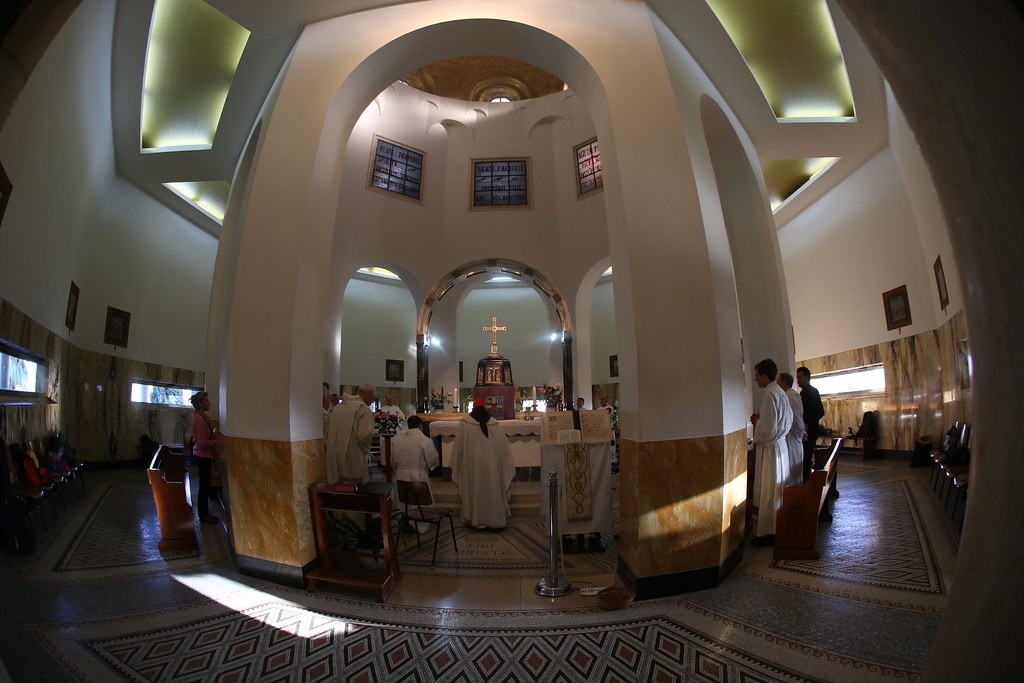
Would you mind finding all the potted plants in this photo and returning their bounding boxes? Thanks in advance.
[335,509,410,556]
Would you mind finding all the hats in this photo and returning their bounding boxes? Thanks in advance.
[473,396,486,408]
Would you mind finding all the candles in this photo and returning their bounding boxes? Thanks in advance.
[532,385,537,405]
[453,388,458,407]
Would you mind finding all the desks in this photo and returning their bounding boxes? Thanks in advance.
[429,420,552,471]
[814,435,879,456]
[306,477,400,601]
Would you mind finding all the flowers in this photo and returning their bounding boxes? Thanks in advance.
[424,387,452,407]
[538,384,562,407]
[375,411,401,436]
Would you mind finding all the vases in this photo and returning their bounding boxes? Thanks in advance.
[546,403,558,413]
[382,425,395,434]
[435,406,445,412]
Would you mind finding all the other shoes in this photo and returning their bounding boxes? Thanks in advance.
[750,535,775,548]
[201,514,220,523]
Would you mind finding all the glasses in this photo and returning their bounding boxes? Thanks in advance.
[753,374,761,380]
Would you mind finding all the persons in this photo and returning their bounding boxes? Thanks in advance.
[190,391,220,524]
[451,398,516,528]
[390,415,439,533]
[776,372,808,487]
[796,367,824,475]
[382,394,405,420]
[749,359,794,546]
[577,398,585,410]
[596,395,613,414]
[323,384,384,549]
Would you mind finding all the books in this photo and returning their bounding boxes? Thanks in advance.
[332,477,363,492]
[544,410,612,443]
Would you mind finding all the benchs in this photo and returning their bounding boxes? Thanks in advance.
[148,443,197,548]
[776,438,845,560]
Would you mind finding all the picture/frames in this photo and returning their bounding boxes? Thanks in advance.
[104,305,131,348]
[0,161,13,228]
[932,253,953,311]
[66,281,80,332]
[384,358,405,382]
[609,355,619,377]
[881,284,913,331]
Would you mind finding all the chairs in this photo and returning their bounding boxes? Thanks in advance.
[930,419,981,518]
[9,433,87,541]
[397,480,459,565]
